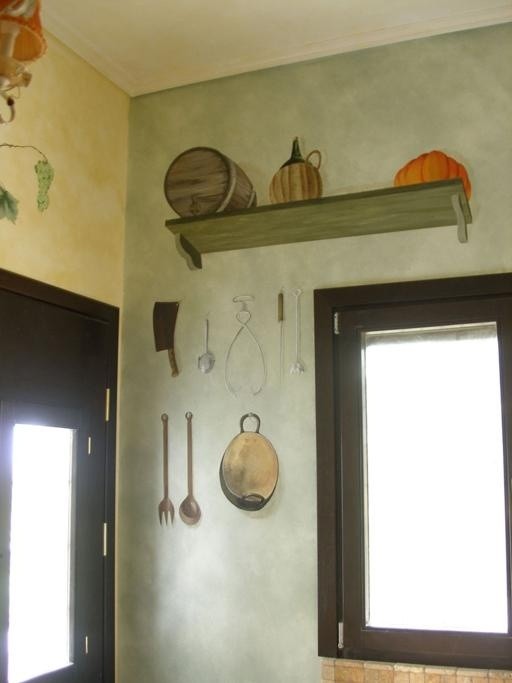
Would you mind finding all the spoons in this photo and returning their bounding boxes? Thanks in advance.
[193,318,218,374]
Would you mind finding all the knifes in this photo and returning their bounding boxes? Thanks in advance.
[151,301,180,377]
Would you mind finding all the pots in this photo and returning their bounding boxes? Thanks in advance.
[220,412,279,511]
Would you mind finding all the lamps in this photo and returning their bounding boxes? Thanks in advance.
[0,1,49,129]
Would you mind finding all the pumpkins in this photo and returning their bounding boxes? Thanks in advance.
[394,151,471,203]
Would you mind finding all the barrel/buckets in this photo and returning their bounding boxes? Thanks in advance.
[162,145,258,220]
